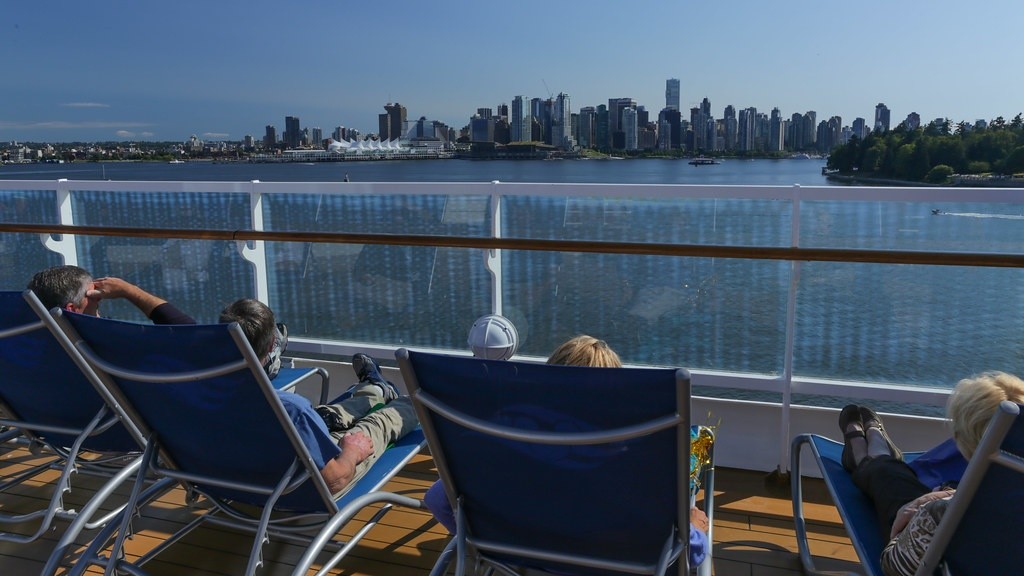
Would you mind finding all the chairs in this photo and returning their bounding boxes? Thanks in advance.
[0,289,717,576]
[790,401,1024,576]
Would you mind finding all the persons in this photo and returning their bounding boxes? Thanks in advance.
[25,265,197,325]
[838,369,1024,576]
[216,296,419,512]
[423,334,709,571]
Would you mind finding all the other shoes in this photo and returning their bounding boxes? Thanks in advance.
[859,405,904,462]
[838,404,863,470]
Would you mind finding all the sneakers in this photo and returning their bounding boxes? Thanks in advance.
[352,352,400,404]
[263,322,288,381]
